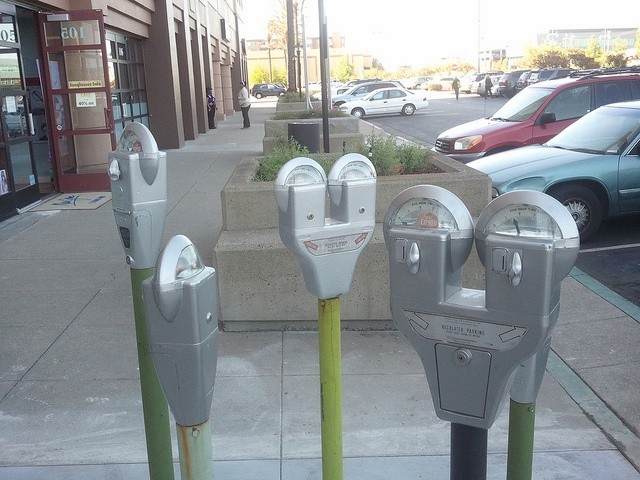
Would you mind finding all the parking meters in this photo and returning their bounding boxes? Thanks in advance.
[142,235,219,480]
[275,153,376,480]
[381,185,579,480]
[108,123,174,480]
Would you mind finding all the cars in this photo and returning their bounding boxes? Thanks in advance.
[460,73,477,93]
[339,87,428,118]
[466,101,640,243]
[407,76,433,90]
[314,87,367,101]
[422,75,455,91]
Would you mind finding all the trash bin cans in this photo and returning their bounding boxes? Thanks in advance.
[288,122,320,154]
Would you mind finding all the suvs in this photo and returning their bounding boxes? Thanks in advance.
[332,81,405,109]
[527,68,578,86]
[472,71,505,97]
[250,84,286,99]
[431,66,640,163]
[499,70,533,98]
[516,70,530,93]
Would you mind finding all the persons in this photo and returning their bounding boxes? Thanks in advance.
[237,81,252,128]
[206,88,218,129]
[485,75,494,99]
[451,76,460,100]
